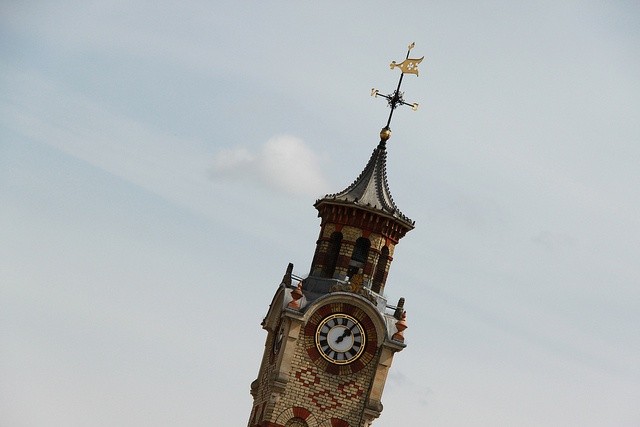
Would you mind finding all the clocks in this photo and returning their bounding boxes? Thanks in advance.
[314,313,368,365]
